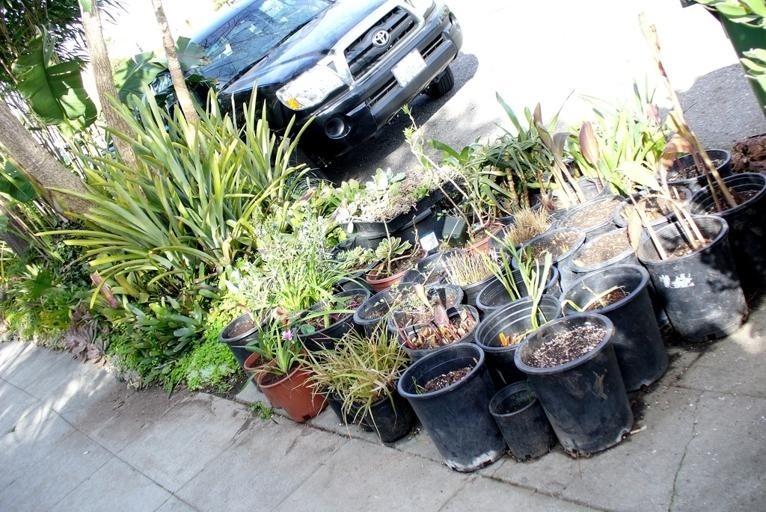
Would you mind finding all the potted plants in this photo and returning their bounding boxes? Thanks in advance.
[184,0,766,472]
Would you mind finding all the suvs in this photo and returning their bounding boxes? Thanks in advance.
[142,0,464,180]
